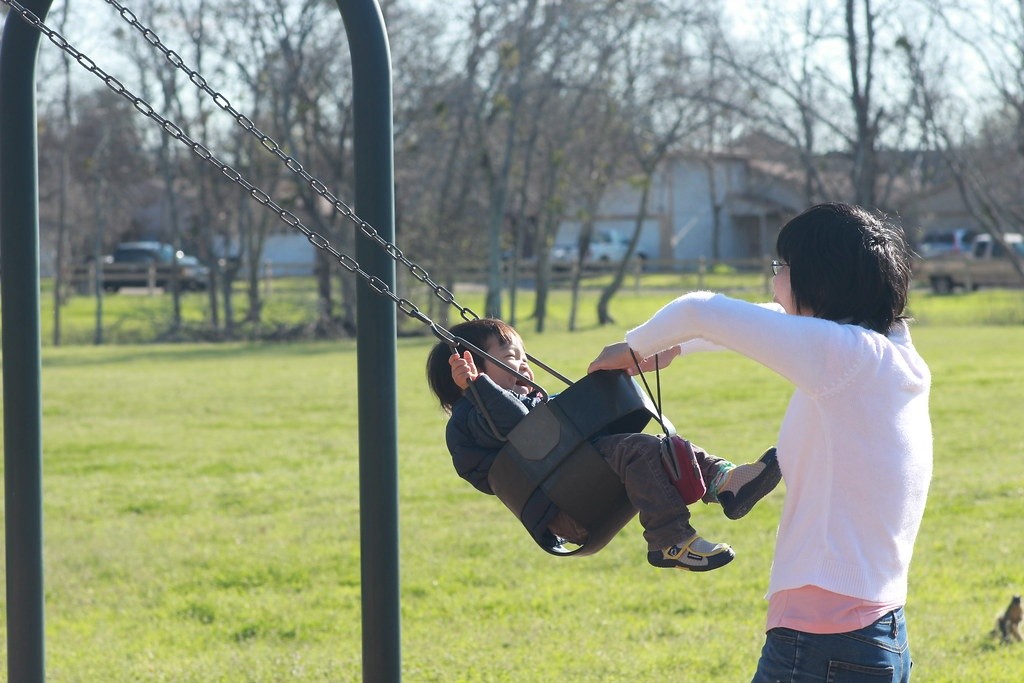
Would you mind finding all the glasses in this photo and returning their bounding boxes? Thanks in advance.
[771,259,789,275]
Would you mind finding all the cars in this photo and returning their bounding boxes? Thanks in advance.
[920,227,973,252]
[552,226,638,270]
[100,240,209,293]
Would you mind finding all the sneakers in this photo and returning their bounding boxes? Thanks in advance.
[647,532,732,573]
[715,444,780,520]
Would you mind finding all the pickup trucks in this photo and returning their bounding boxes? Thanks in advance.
[921,232,1024,296]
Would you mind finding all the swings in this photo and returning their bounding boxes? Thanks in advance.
[2,0,677,560]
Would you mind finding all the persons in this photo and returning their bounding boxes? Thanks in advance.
[424,316,787,572]
[587,200,934,683]
